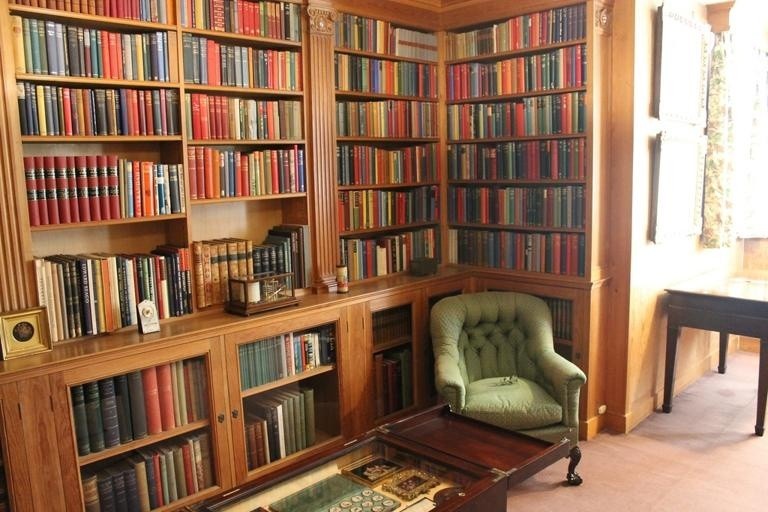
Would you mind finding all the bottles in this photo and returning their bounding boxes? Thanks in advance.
[336,265,348,294]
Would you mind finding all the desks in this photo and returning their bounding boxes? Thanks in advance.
[660,282,768,437]
[170,405,576,512]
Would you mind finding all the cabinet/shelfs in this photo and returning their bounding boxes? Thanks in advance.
[359,277,471,423]
[328,1,444,285]
[176,0,329,311]
[1,1,178,353]
[0,303,357,512]
[471,280,608,439]
[443,1,612,278]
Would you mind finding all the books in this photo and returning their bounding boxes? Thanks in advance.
[5,2,590,510]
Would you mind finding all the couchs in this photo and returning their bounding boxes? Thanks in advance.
[433,292,586,489]
